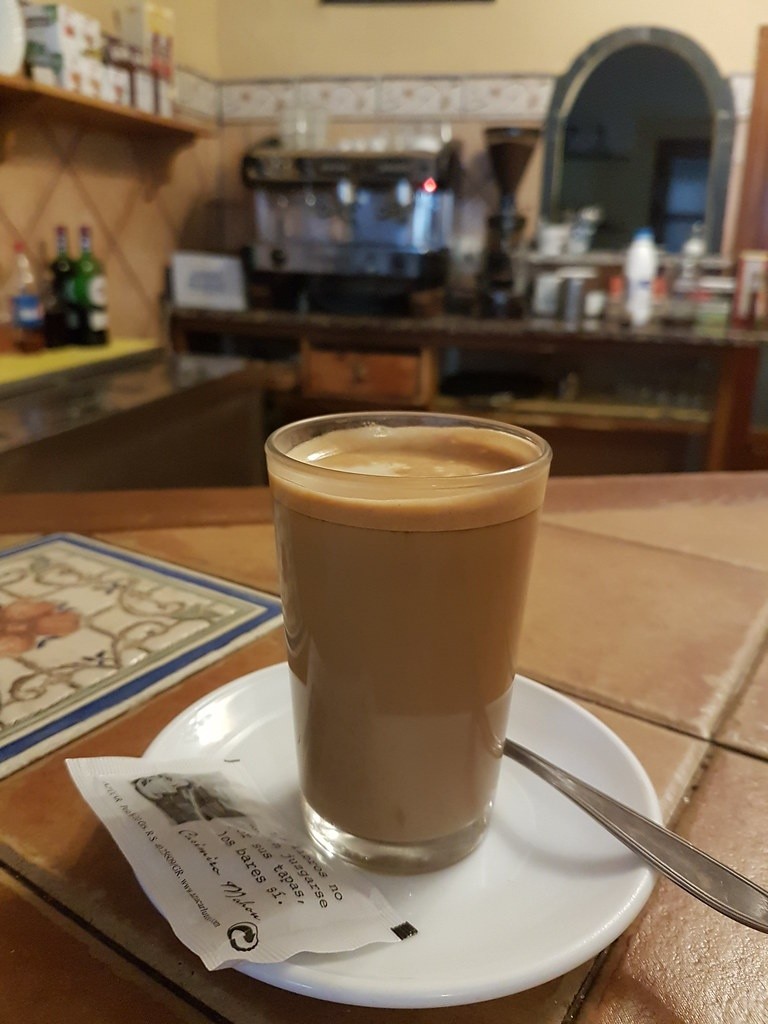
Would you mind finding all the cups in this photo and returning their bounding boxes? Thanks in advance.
[261,410,555,874]
[561,276,586,321]
[530,275,564,318]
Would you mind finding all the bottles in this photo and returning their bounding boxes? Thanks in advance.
[34,241,68,347]
[72,227,110,346]
[9,243,47,351]
[51,226,76,340]
[623,225,710,329]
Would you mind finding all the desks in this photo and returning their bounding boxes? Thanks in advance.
[1,470,768,1024]
[157,296,768,471]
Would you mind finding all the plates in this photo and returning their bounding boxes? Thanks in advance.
[136,661,664,1011]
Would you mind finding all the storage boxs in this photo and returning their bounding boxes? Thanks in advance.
[25,2,180,118]
